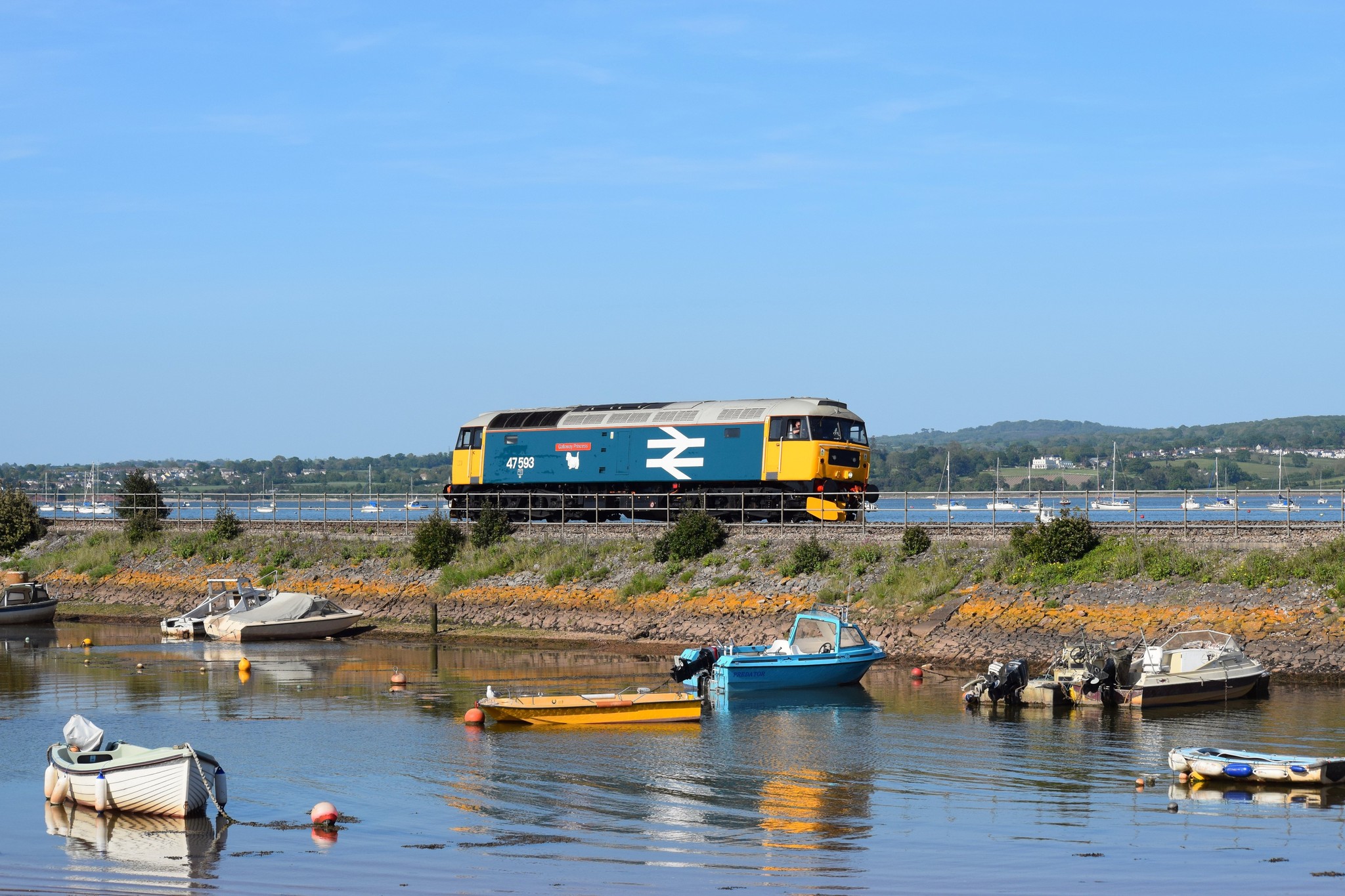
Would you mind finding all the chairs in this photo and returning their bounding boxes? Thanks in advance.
[790,644,811,656]
[766,640,792,656]
[1142,646,1171,674]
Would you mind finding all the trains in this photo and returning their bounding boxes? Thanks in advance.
[441,396,880,525]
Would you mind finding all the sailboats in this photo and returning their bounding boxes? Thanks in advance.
[1019,461,1052,511]
[256,472,275,512]
[1091,441,1131,509]
[986,457,1017,510]
[362,464,387,514]
[1059,472,1072,505]
[442,475,453,510]
[932,450,968,511]
[1317,471,1328,504]
[39,460,111,514]
[404,476,429,510]
[1202,456,1239,509]
[1267,449,1300,512]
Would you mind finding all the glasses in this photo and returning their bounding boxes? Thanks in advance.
[796,424,800,426]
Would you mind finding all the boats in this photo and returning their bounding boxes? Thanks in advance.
[160,570,281,636]
[1181,499,1200,509]
[1167,783,1345,807]
[1170,745,1345,785]
[479,692,704,725]
[0,579,61,625]
[203,591,364,643]
[1070,630,1266,708]
[673,603,888,695]
[47,740,219,820]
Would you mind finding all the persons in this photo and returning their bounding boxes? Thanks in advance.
[792,421,805,438]
